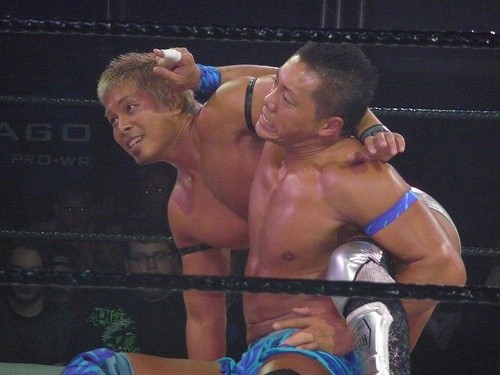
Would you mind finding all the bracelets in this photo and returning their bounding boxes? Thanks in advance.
[360,124,392,145]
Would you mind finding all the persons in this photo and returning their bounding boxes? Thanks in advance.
[46,244,91,320]
[97,227,188,359]
[61,41,466,375]
[0,241,96,364]
[97,41,461,375]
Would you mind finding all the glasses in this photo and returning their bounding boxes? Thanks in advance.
[126,250,173,263]
